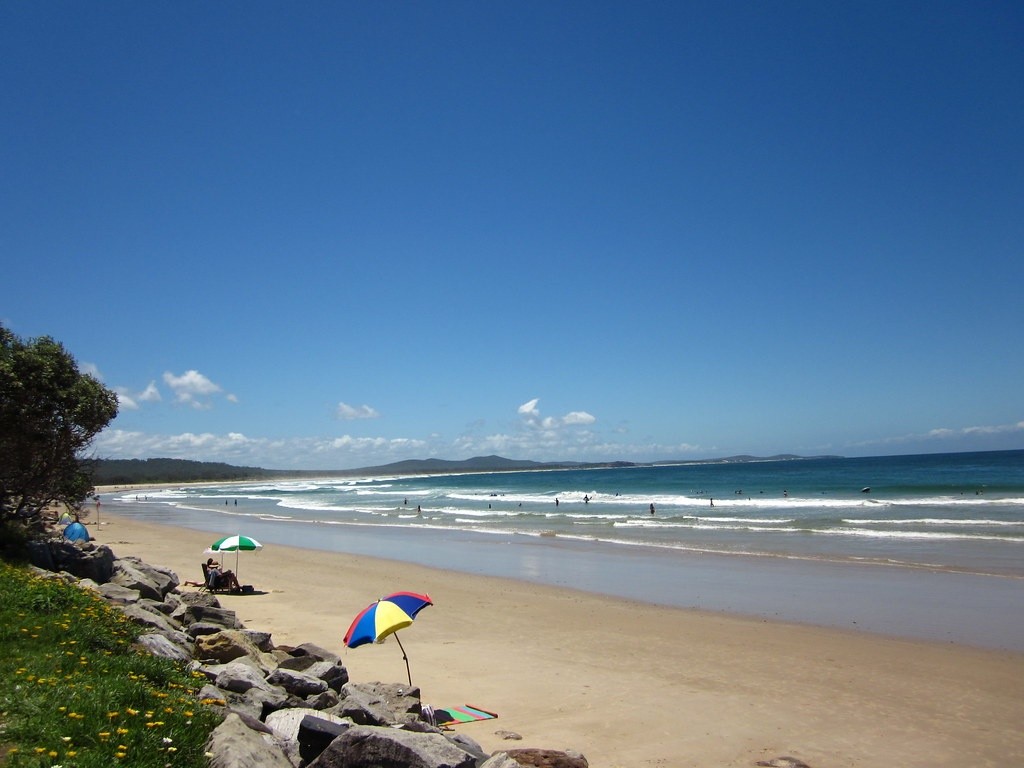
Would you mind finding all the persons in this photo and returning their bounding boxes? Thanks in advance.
[207,558,243,588]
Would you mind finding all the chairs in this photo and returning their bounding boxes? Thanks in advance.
[201,563,233,593]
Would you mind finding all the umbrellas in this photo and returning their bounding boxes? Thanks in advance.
[204,535,263,580]
[343,592,433,687]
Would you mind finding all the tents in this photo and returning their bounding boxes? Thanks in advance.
[59,512,72,525]
[62,521,89,543]
